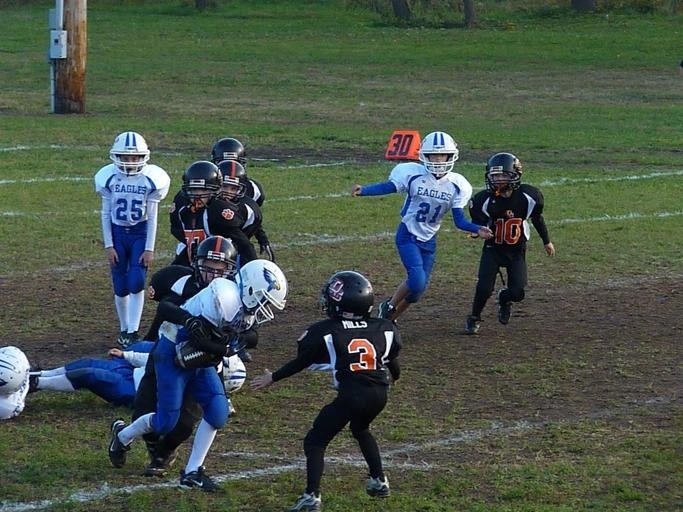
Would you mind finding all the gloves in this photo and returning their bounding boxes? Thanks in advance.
[185,316,208,338]
[224,337,248,358]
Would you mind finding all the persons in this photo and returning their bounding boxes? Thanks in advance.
[250,271,401,507]
[466,154,555,336]
[28,348,246,407]
[352,131,492,327]
[216,160,274,261]
[95,130,171,347]
[171,160,275,269]
[177,136,259,207]
[146,237,236,340]
[108,257,287,494]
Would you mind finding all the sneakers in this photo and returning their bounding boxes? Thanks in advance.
[497,288,513,325]
[117,330,127,345]
[27,375,41,394]
[238,349,252,362]
[365,475,390,498]
[377,296,397,320]
[285,491,322,512]
[121,331,139,348]
[464,314,481,335]
[108,417,224,494]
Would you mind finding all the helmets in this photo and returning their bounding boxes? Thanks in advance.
[191,235,239,288]
[233,259,287,325]
[217,354,247,395]
[483,152,523,195]
[181,138,250,212]
[108,132,149,176]
[416,131,459,178]
[319,271,374,321]
[0,346,30,419]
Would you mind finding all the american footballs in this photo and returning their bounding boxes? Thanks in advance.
[175,343,219,369]
